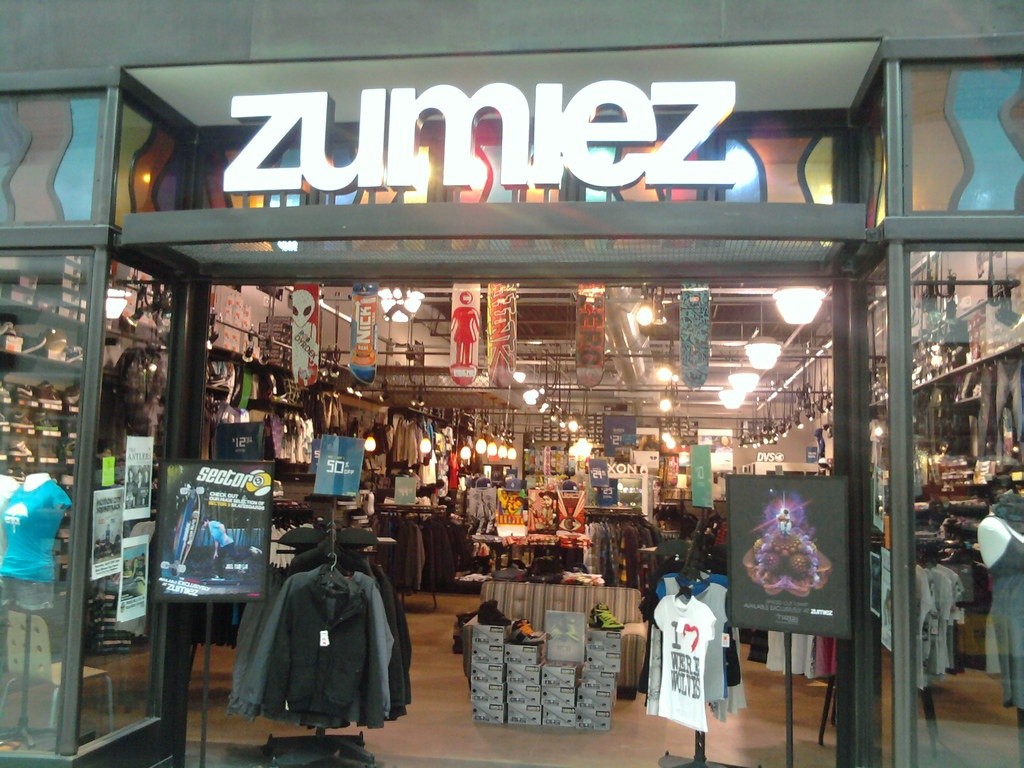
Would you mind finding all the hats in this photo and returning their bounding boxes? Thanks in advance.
[563,481,577,491]
[503,478,522,492]
[477,477,490,488]
[538,491,559,501]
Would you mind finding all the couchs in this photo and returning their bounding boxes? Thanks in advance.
[462,581,648,701]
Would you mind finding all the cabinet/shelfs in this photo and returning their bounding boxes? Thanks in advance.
[870,477,1010,573]
[0,304,121,487]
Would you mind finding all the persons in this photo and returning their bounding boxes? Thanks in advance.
[0,471,73,608]
[978,490,1024,767]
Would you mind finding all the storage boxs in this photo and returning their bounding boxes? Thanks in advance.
[259,316,293,368]
[0,253,113,331]
[470,624,622,732]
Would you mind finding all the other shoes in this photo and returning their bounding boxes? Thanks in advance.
[491,563,528,582]
[550,616,581,644]
[251,546,262,554]
[477,600,513,626]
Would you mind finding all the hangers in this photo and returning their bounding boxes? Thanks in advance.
[675,547,710,599]
[319,521,350,590]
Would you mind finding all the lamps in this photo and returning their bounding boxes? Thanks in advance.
[208,319,427,409]
[518,345,580,432]
[718,387,745,409]
[116,279,173,328]
[727,370,761,393]
[460,394,517,460]
[378,286,425,323]
[743,337,782,370]
[771,287,822,324]
[738,354,832,449]
[419,407,432,452]
[911,250,1023,347]
[364,391,377,452]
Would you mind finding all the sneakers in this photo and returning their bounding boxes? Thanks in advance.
[0,321,82,611]
[588,603,625,630]
[510,619,551,645]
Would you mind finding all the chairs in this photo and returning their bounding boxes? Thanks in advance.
[0,610,116,732]
